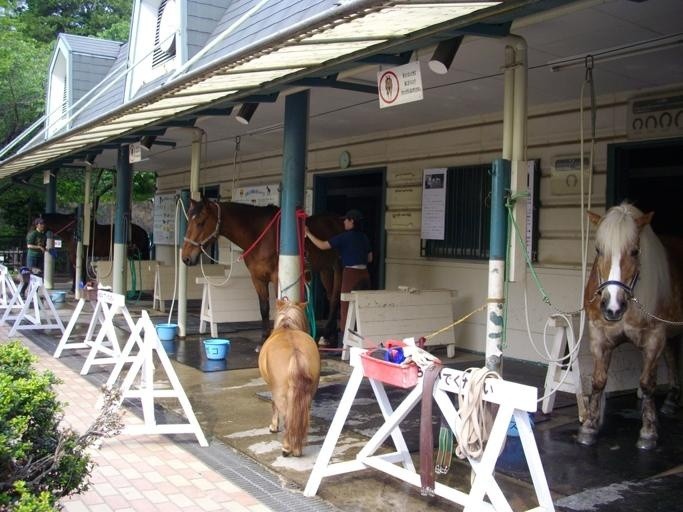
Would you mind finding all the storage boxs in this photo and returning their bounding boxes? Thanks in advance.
[360,350,419,389]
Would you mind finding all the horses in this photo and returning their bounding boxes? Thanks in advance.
[257,297,321,458]
[181,192,345,353]
[26,211,151,296]
[576,200,683,451]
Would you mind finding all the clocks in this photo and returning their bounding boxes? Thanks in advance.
[338,151,351,170]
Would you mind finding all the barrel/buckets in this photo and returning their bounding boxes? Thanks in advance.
[509,412,535,436]
[160,341,176,354]
[203,339,232,360]
[207,360,226,371]
[156,324,178,341]
[52,292,67,302]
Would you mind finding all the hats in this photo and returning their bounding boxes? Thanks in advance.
[339,209,365,223]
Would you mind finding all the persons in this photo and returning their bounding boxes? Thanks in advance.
[25,217,47,274]
[305,209,373,336]
